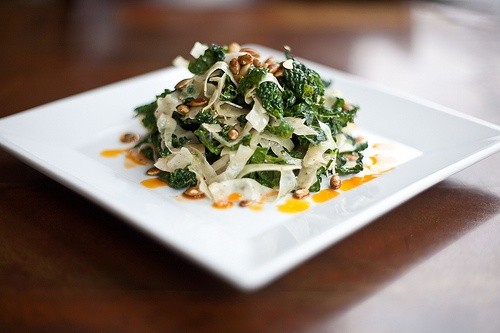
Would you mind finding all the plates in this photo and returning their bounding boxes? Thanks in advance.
[0,42,500,292]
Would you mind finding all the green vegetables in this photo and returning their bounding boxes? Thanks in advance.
[130,43,369,202]
